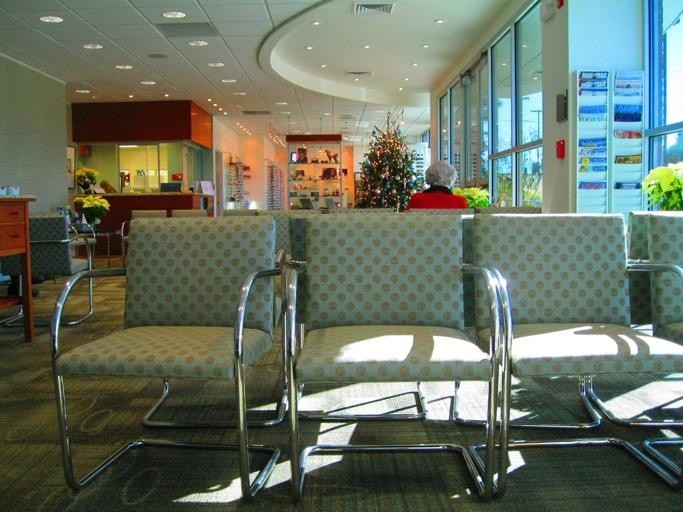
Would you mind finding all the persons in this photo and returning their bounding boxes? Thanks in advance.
[405,161,468,209]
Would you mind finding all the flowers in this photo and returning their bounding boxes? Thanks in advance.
[74,167,99,191]
[450,185,492,208]
[641,162,683,212]
[73,196,111,228]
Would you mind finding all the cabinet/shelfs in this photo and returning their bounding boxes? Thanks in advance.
[286,138,343,209]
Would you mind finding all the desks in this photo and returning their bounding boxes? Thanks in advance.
[0,194,38,343]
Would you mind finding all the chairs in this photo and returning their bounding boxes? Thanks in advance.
[5,214,111,329]
[474,212,683,498]
[119,207,683,430]
[284,212,499,502]
[50,216,286,500]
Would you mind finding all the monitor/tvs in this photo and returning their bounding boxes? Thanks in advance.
[160,182,181,192]
